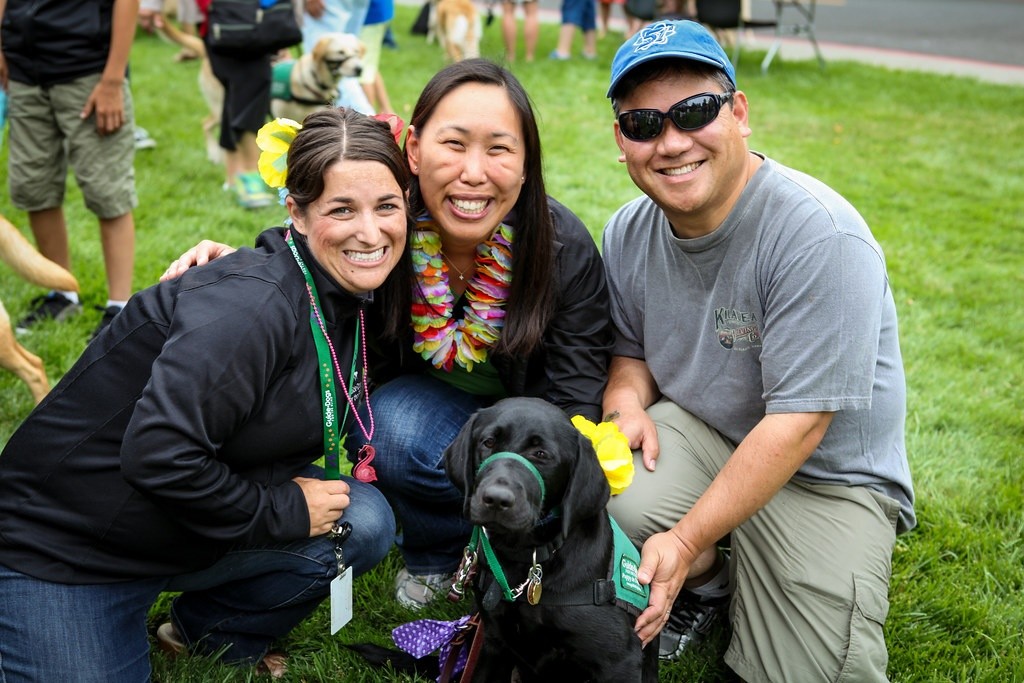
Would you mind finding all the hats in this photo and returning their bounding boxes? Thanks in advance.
[606,18,736,102]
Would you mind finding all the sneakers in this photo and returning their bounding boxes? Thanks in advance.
[659,586,731,659]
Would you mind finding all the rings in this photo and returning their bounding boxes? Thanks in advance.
[667,610,671,613]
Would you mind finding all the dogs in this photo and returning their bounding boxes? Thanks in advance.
[427,0,481,63]
[445,397,660,683]
[150,14,366,169]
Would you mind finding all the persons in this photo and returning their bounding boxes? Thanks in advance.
[132,0,756,207]
[157,55,612,609]
[598,18,917,683]
[0,105,415,683]
[1,0,136,351]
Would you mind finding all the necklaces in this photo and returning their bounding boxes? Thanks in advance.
[410,206,514,374]
[279,222,377,484]
[443,251,475,282]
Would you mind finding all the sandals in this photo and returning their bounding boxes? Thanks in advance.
[158,620,292,680]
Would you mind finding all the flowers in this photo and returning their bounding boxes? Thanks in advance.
[374,112,405,143]
[572,415,634,497]
[256,118,303,190]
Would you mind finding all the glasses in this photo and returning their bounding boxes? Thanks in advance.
[619,92,735,142]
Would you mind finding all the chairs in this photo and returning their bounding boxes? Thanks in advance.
[695,0,826,76]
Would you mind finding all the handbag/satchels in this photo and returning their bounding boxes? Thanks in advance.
[206,1,304,58]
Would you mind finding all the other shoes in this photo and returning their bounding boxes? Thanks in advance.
[13,289,79,335]
[133,123,158,152]
[393,568,455,612]
[86,304,119,346]
[232,174,269,209]
